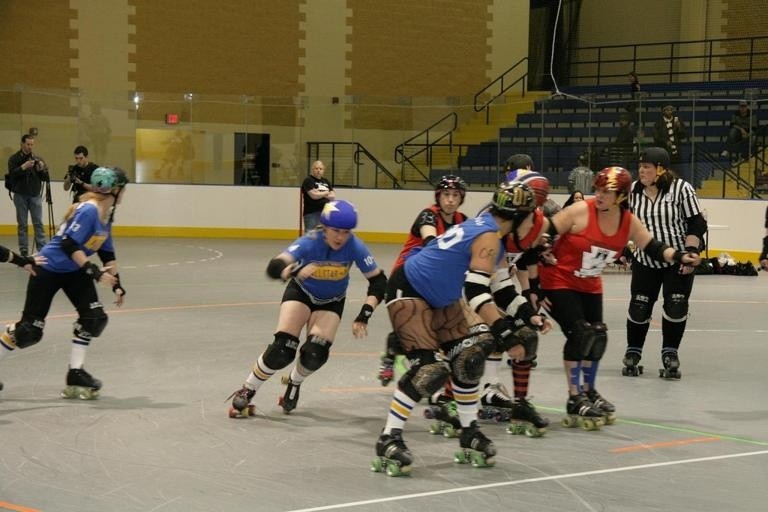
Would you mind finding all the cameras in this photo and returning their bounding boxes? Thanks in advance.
[69,165,78,183]
[31,160,40,165]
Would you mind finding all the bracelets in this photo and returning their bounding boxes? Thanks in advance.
[353,302,373,324]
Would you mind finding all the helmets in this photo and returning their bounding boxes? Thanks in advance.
[320,199,357,229]
[591,166,631,194]
[505,154,535,172]
[90,166,129,193]
[638,146,671,170]
[491,180,536,213]
[434,175,466,205]
[499,169,549,206]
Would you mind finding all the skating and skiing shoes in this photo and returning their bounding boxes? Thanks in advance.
[658,349,683,379]
[424,400,463,438]
[505,400,549,438]
[59,367,101,400]
[561,392,607,431]
[581,385,617,425]
[454,420,497,467]
[370,427,414,477]
[228,384,256,418]
[479,383,513,421]
[277,370,300,414]
[621,350,644,377]
[377,351,395,386]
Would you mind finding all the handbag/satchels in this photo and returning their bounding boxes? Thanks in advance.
[3,173,41,198]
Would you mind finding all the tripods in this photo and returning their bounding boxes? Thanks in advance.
[31,170,55,255]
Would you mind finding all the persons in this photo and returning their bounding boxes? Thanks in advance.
[653,103,690,167]
[224,195,388,424]
[299,159,338,235]
[758,204,768,275]
[0,166,131,389]
[0,244,51,396]
[566,155,596,196]
[719,99,758,163]
[625,71,641,113]
[3,133,51,258]
[60,145,100,204]
[611,114,636,164]
[633,129,649,158]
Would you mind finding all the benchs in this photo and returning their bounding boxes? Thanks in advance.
[425,71,767,200]
